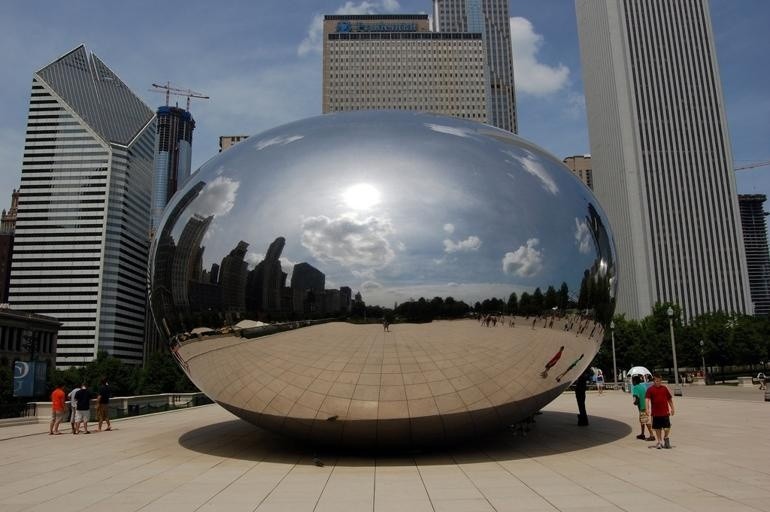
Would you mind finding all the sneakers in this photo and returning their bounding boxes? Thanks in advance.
[646,436,655,440]
[637,434,645,440]
[657,442,664,449]
[664,438,671,448]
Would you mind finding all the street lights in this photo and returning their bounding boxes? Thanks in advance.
[700,340,706,376]
[666,306,679,384]
[610,321,617,383]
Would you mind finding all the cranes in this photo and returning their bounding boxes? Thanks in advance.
[734,159,770,172]
[149,81,210,111]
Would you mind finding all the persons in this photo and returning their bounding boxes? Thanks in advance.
[74,383,89,435]
[49,382,67,434]
[68,384,81,432]
[95,376,114,433]
[467,311,603,382]
[596,369,605,394]
[631,373,675,449]
[383,319,389,332]
[572,375,590,426]
[757,370,766,390]
